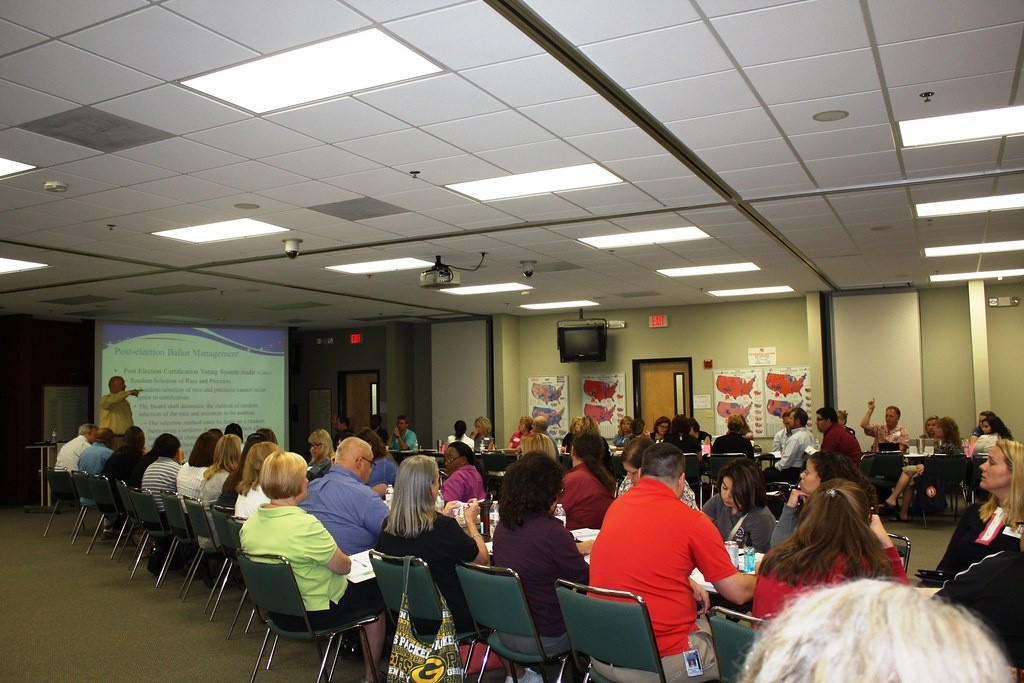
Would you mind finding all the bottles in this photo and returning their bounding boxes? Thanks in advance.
[744,536,756,575]
[51,429,57,443]
[701,436,712,457]
[557,439,562,457]
[813,439,822,451]
[480,440,484,454]
[489,501,499,540]
[478,500,492,536]
[960,432,979,457]
[435,490,444,513]
[554,504,567,528]
[441,440,446,453]
[386,484,394,510]
[413,440,418,451]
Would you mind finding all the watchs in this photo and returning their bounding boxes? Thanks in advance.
[396,434,400,438]
[178,461,186,465]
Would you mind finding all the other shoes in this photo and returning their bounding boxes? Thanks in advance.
[506,668,548,683]
[101,527,119,540]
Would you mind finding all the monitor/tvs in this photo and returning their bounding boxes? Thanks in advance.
[558,326,607,362]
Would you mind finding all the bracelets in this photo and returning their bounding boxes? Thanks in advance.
[471,534,483,538]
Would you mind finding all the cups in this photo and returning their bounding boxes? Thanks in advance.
[916,438,925,454]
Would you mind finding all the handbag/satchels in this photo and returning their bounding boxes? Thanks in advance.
[387,556,468,683]
[133,530,156,558]
[147,546,184,575]
[913,477,948,516]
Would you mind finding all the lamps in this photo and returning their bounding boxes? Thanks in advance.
[989,297,1020,308]
[316,337,333,345]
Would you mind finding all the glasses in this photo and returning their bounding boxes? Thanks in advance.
[356,456,377,470]
[310,442,323,449]
[445,456,460,462]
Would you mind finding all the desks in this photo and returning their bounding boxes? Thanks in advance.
[25,445,65,514]
[389,450,444,460]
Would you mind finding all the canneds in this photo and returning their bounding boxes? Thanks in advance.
[724,541,739,569]
[440,444,445,453]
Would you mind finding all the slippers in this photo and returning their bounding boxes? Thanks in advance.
[890,514,910,522]
[878,500,897,509]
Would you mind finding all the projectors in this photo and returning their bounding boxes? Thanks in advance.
[420,271,461,289]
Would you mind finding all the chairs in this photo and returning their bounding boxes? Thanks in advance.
[39,451,989,683]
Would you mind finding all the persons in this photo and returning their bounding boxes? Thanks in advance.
[331,396,1013,522]
[54,423,1024,683]
[99,376,143,451]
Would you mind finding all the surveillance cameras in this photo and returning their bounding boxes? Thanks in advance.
[522,262,534,277]
[284,240,299,258]
